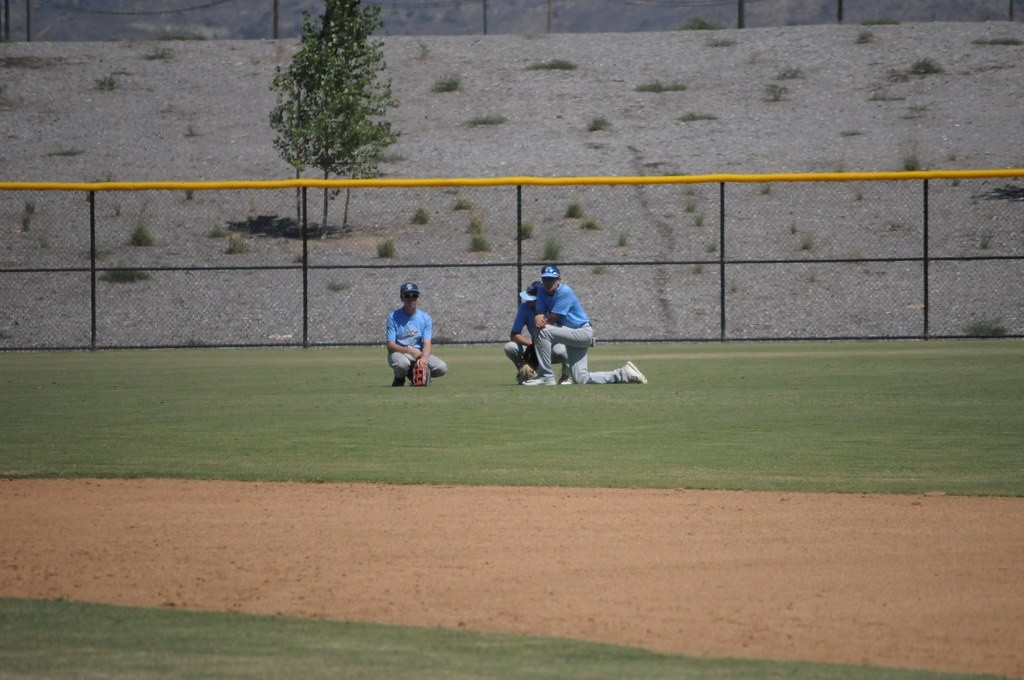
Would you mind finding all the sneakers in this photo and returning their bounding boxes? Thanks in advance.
[522,376,556,386]
[623,360,648,384]
[558,373,574,384]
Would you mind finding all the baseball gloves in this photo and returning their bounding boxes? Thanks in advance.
[516,359,539,385]
[411,359,431,386]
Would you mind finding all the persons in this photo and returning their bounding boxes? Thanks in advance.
[386,283,448,387]
[504,281,575,385]
[521,264,648,386]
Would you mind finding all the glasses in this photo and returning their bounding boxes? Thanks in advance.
[403,294,419,299]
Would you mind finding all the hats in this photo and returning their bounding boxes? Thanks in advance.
[519,281,542,301]
[400,282,420,296]
[540,264,560,277]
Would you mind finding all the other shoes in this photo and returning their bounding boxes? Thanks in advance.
[391,376,406,387]
